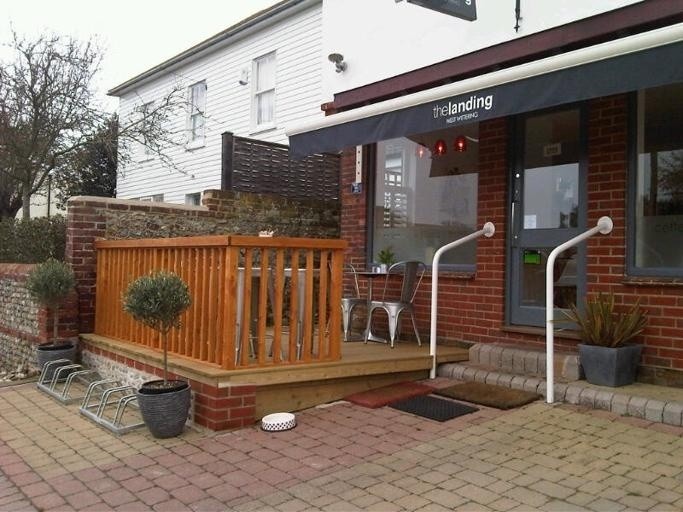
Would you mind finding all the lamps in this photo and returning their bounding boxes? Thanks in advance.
[413,128,477,157]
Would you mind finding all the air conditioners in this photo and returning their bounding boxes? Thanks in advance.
[375,245,396,273]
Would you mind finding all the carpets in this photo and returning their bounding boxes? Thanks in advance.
[345,377,542,422]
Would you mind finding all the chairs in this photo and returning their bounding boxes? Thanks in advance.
[325,260,365,342]
[364,261,427,349]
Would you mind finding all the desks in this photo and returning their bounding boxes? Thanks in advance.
[345,268,398,344]
[215,266,323,365]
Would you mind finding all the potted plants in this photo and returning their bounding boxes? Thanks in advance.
[550,289,649,387]
[28,257,81,380]
[120,267,191,437]
